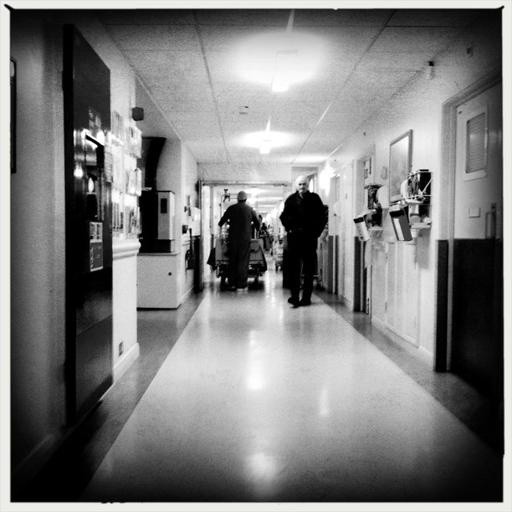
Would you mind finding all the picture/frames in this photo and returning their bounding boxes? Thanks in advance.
[388,129,412,206]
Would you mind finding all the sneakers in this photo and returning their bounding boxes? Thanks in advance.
[288,296,299,304]
[293,299,312,308]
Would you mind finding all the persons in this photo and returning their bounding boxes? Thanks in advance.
[280,175,326,307]
[219,192,261,292]
